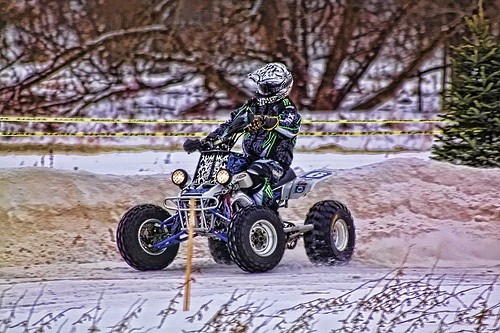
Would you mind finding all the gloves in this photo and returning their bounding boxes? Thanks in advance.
[183,138,203,153]
[246,114,263,133]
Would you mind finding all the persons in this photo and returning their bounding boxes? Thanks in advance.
[183,62,303,216]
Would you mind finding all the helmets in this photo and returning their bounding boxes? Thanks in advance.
[247,62,294,106]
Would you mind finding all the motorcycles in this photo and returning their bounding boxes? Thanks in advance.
[116,114,357,272]
